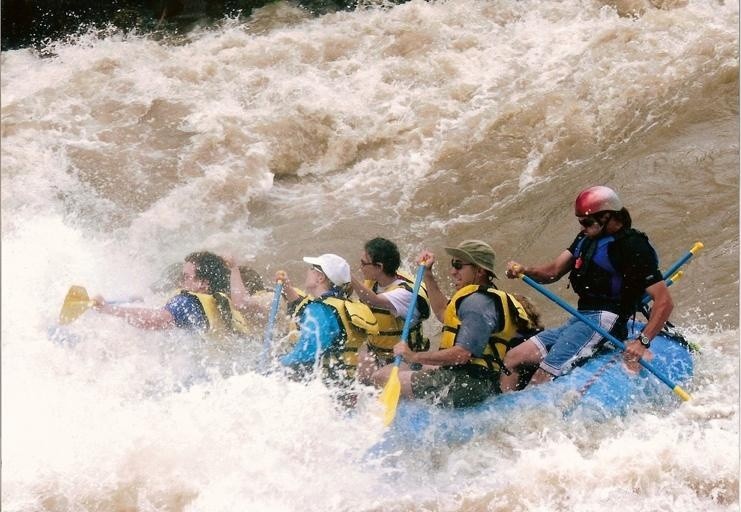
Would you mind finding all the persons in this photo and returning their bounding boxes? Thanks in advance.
[347,238,501,409]
[496,183,677,393]
[95,250,361,384]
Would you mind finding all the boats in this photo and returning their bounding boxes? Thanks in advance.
[45,312,722,469]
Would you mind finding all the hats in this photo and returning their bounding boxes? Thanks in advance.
[445,239,498,279]
[302,253,350,286]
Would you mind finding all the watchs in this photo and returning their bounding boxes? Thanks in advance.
[639,332,651,350]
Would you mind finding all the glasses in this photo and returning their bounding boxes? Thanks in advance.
[311,266,324,274]
[578,217,597,228]
[451,260,473,269]
[360,259,375,267]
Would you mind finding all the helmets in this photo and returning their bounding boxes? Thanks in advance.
[576,187,623,216]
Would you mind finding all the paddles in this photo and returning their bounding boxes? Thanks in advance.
[380,257,426,424]
[57,284,144,324]
